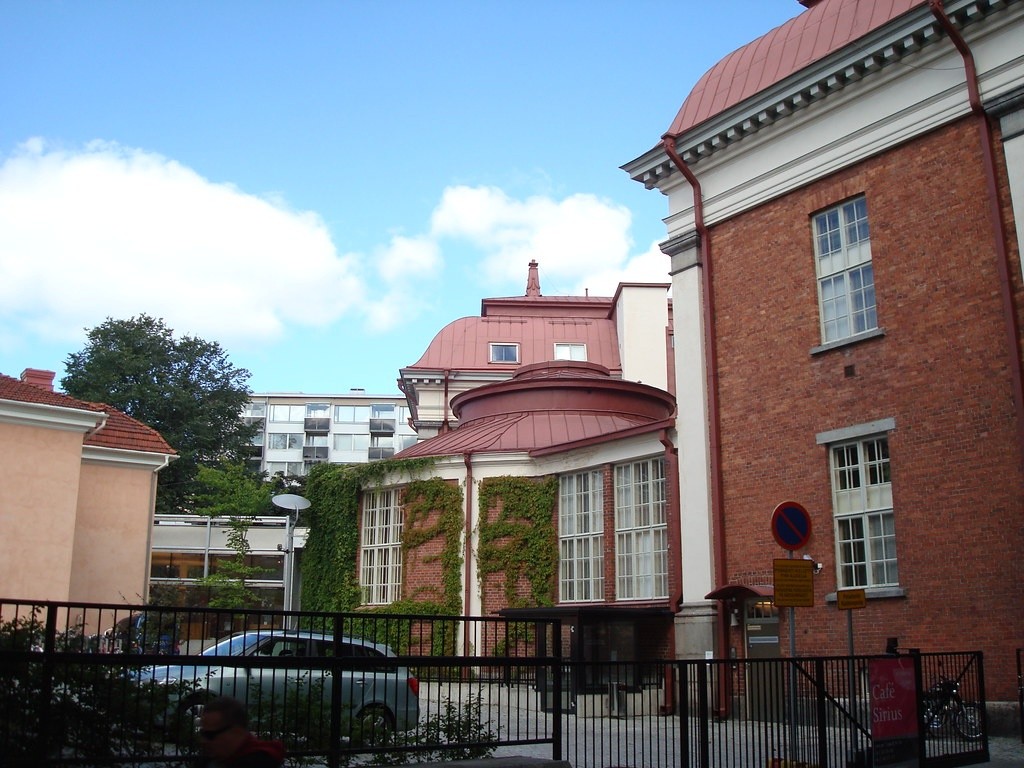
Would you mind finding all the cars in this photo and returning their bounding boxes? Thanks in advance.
[92,613,180,656]
[105,631,420,748]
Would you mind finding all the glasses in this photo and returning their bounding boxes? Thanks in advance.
[199,717,237,741]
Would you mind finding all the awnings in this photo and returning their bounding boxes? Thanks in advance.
[704,583,775,605]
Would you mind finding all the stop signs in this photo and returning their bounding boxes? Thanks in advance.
[771,501,812,551]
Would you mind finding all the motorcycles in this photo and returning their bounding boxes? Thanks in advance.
[922,662,991,742]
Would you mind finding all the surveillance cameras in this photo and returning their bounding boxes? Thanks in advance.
[277,544,283,550]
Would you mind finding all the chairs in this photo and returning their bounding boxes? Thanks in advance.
[279,647,306,656]
